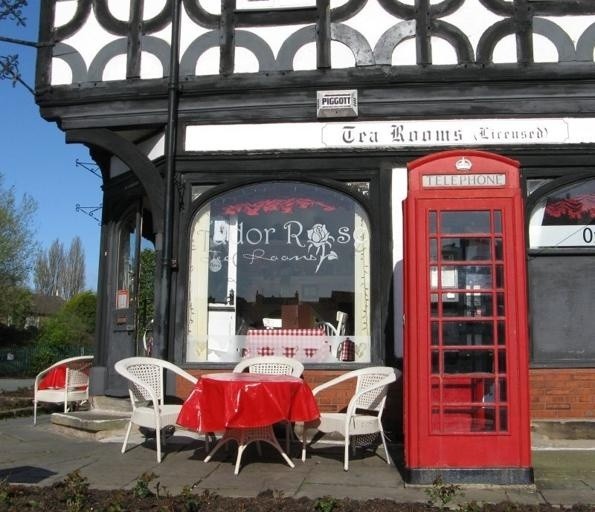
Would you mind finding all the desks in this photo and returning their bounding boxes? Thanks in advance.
[193,372,314,475]
[242,326,327,365]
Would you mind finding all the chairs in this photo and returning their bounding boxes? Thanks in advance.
[301,365,404,472]
[325,311,348,365]
[32,353,92,425]
[224,357,306,457]
[114,357,212,463]
[262,317,283,329]
